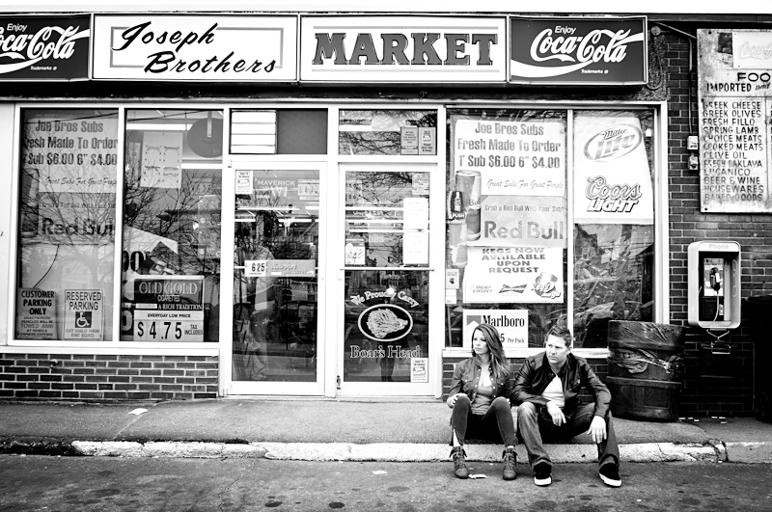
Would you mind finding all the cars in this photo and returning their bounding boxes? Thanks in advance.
[249,282,371,372]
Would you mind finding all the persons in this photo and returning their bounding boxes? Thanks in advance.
[232,225,278,381]
[445,322,520,481]
[507,323,624,488]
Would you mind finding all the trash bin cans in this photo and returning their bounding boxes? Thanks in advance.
[607,320,686,422]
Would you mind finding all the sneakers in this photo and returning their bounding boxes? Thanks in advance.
[502,450,518,480]
[533,463,553,486]
[599,463,622,487]
[449,448,468,478]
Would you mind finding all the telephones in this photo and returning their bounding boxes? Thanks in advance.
[704,257,724,298]
